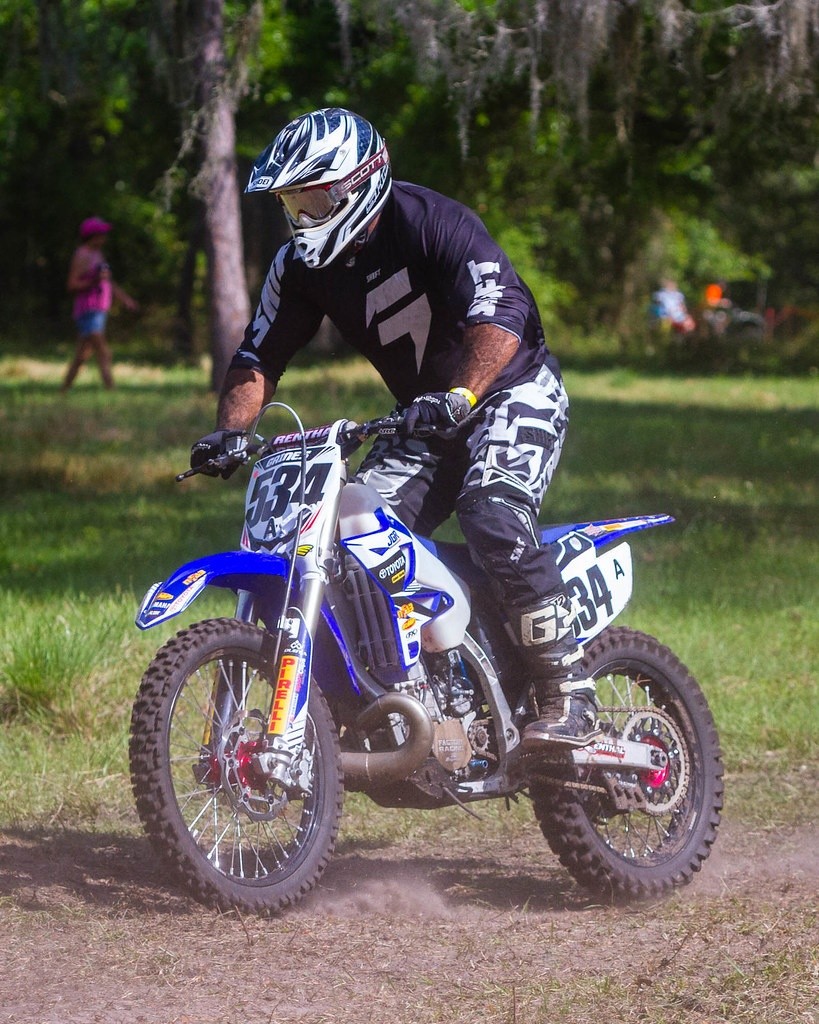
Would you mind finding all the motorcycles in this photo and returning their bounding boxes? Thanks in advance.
[128,399,724,916]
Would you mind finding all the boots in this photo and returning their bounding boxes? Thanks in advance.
[504,592,602,752]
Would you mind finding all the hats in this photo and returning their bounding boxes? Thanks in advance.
[80,216,112,237]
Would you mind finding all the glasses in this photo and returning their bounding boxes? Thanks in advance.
[274,183,341,228]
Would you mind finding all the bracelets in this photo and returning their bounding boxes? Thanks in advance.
[449,388,477,408]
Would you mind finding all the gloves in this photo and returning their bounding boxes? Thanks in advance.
[190,431,252,480]
[404,386,477,440]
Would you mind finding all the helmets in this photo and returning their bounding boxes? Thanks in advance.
[244,108,392,269]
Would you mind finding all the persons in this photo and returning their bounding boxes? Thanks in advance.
[59,217,136,390]
[191,109,602,747]
[648,279,686,341]
